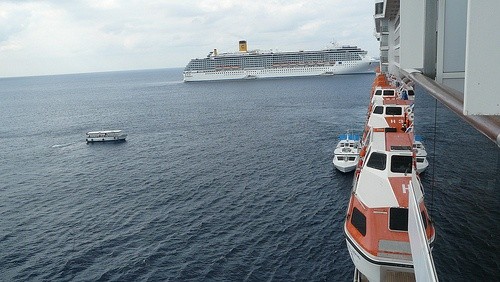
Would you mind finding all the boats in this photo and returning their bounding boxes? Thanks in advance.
[333,130,361,173]
[414,134,430,173]
[343,65,435,282]
[84,130,127,143]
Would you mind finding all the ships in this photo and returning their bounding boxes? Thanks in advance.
[184,40,372,78]
